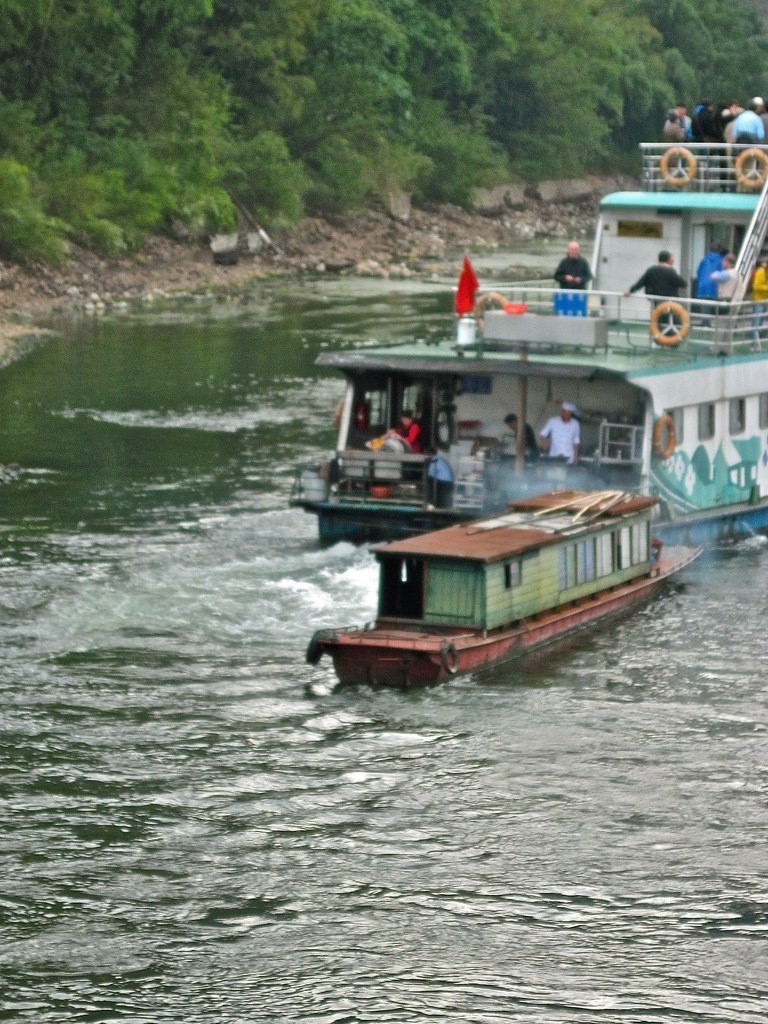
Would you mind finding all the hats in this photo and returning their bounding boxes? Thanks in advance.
[562,401,577,411]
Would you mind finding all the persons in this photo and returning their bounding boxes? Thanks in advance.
[662,96,768,192]
[539,402,580,466]
[364,410,428,455]
[423,446,455,511]
[553,242,592,291]
[695,238,768,352]
[504,413,538,461]
[625,251,687,350]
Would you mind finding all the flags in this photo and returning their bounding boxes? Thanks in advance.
[453,256,479,316]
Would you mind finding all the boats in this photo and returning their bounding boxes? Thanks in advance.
[305,489,705,687]
[291,144,768,559]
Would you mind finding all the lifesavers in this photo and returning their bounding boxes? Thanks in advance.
[306,629,329,666]
[649,300,691,346]
[659,146,698,186]
[653,413,678,459]
[735,147,768,189]
[473,291,508,328]
[440,643,459,675]
